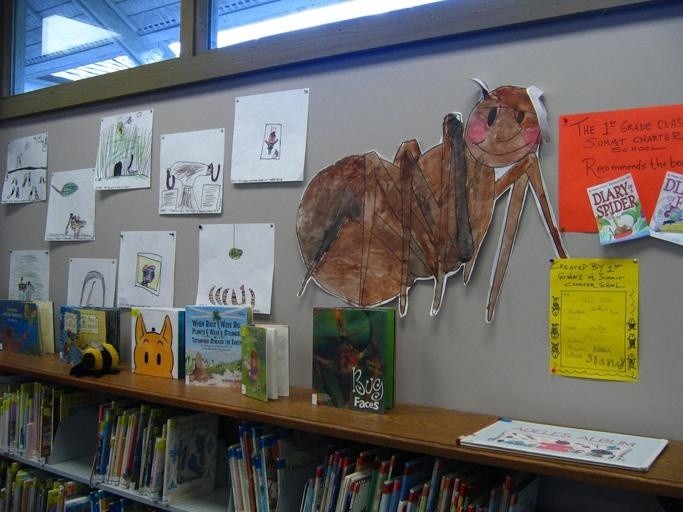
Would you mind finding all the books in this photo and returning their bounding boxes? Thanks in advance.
[59,306,120,367]
[227,427,539,512]
[311,306,396,414]
[456,418,669,472]
[131,304,289,402]
[2,374,219,511]
[1,300,45,358]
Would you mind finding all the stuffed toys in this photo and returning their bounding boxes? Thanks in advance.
[69,344,120,380]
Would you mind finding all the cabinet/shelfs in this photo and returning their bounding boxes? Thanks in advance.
[0,352,682,511]
